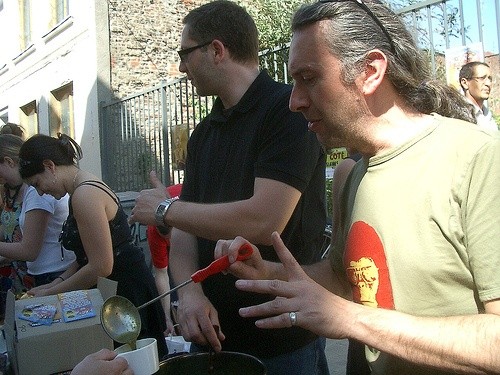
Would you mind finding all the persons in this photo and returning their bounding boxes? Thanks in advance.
[0,121,41,329]
[213,0,500,375]
[128,0,334,375]
[0,134,79,290]
[69,347,137,375]
[20,135,171,362]
[147,184,184,338]
[443,62,500,136]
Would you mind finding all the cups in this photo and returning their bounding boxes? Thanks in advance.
[110,338,160,375]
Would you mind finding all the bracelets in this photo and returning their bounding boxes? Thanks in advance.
[56,277,65,281]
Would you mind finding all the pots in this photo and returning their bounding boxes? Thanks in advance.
[158,351,266,375]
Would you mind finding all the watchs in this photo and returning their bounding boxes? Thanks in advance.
[154,198,177,231]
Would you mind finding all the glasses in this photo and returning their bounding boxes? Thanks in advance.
[177,41,233,62]
[465,76,492,82]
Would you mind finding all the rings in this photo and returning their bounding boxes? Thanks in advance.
[289,311,297,327]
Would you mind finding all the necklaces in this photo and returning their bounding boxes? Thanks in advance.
[3,183,22,209]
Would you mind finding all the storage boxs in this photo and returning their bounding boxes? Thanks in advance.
[5,277,118,375]
